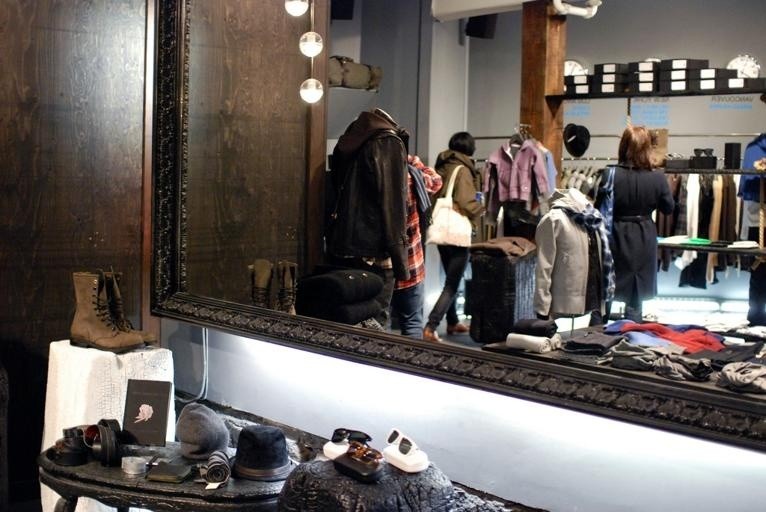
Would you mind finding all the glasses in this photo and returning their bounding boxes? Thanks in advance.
[386,430,415,457]
[329,426,384,466]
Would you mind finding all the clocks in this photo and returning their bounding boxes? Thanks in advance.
[726,55,760,78]
[564,59,585,76]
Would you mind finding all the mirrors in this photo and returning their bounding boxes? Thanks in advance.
[150,1,766,453]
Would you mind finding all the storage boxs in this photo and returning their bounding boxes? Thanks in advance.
[564,59,766,94]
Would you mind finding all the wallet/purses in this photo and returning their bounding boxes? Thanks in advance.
[146,460,192,484]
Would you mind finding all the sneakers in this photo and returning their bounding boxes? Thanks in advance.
[445,321,471,337]
[422,328,444,342]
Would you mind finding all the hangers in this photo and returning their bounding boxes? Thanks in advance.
[561,155,611,178]
[510,126,532,144]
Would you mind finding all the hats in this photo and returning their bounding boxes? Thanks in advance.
[562,123,590,158]
[174,402,228,460]
[228,424,297,483]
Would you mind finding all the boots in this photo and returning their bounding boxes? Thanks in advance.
[246,257,299,316]
[68,264,160,354]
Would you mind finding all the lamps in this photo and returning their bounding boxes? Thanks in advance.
[285,0,323,104]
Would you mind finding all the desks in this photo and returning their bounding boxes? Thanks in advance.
[39,442,298,512]
[482,325,766,402]
[654,243,761,255]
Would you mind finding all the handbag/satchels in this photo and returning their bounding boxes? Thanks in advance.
[596,165,615,232]
[424,164,474,249]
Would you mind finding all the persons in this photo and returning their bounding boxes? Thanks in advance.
[588,127,675,326]
[535,186,606,318]
[424,132,488,340]
[391,127,444,338]
[329,107,411,333]
